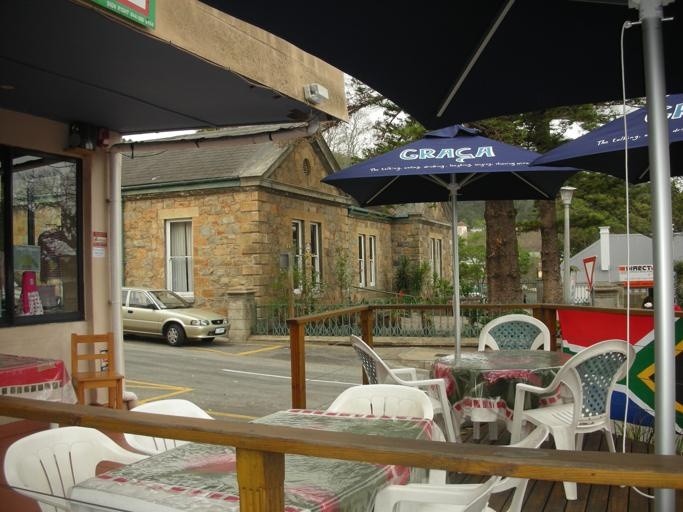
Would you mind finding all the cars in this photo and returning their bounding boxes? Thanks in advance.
[121,286,231,347]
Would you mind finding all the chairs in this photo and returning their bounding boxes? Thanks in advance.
[350,334,460,445]
[511,339,636,502]
[376,474,501,510]
[1,424,149,510]
[121,398,219,455]
[325,382,447,484]
[499,432,549,509]
[472,316,550,439]
[69,332,124,410]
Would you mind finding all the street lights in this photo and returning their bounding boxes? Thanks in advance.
[560,185,576,305]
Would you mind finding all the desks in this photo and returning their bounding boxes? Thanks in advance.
[0,352,64,429]
[429,348,577,425]
[38,409,431,511]
